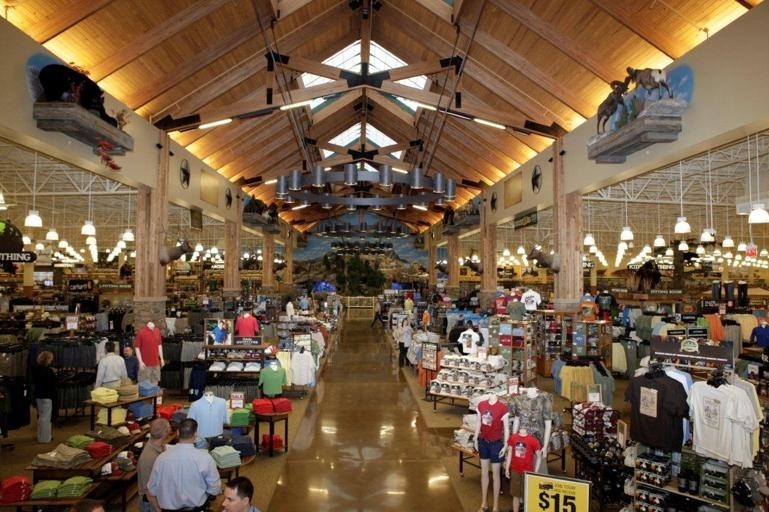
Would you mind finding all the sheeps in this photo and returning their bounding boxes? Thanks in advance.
[626,67,672,100]
[597,77,630,135]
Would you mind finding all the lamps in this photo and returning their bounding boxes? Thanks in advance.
[269,161,457,216]
[0,135,287,268]
[316,218,422,260]
[434,129,769,274]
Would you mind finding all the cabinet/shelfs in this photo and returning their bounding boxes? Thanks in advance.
[1,265,769,512]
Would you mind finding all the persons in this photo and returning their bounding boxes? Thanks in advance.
[133,322,165,385]
[187,392,230,444]
[123,345,139,385]
[373,290,484,370]
[34,351,73,444]
[137,419,171,512]
[210,294,311,400]
[94,341,127,390]
[473,387,554,512]
[222,477,260,511]
[507,290,542,321]
[147,419,222,511]
